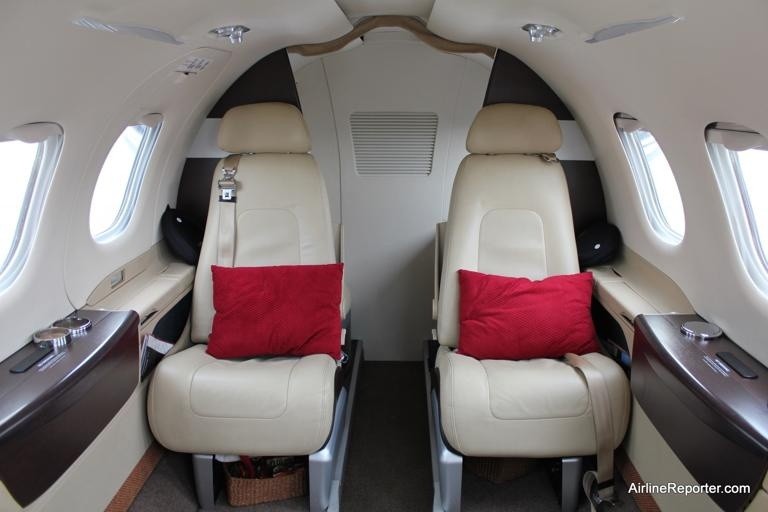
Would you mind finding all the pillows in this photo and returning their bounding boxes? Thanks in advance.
[203,262,347,362]
[455,268,604,359]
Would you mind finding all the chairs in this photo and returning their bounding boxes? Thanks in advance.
[140,101,349,507]
[431,102,634,512]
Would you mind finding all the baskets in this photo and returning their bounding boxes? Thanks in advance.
[224,458,307,508]
